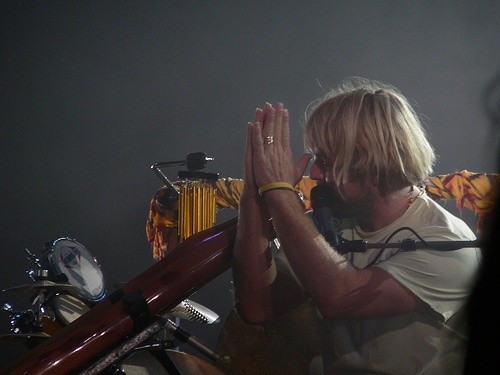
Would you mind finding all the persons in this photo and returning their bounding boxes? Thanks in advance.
[232,80,482,375]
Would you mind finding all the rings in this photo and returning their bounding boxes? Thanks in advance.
[262,136,274,145]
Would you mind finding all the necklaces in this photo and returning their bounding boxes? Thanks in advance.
[408,184,414,205]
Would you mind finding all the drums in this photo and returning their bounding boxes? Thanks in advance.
[52,238,107,303]
[53,291,94,324]
[166,298,220,324]
[116,347,226,375]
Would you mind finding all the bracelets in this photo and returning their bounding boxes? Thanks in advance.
[258,181,294,196]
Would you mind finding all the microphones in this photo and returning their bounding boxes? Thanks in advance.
[159,318,233,373]
[310,185,343,254]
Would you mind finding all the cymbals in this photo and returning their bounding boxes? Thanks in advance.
[2,333,50,339]
[2,281,73,292]
